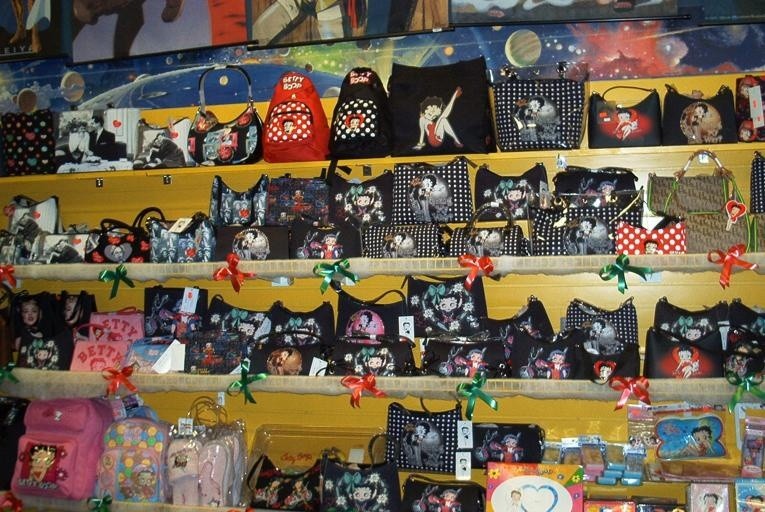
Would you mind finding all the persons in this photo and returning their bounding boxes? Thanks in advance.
[87,116,120,161]
[69,123,88,164]
[110,245,125,261]
[12,300,46,361]
[64,298,86,325]
[14,213,39,237]
[45,239,81,262]
[9,0,53,50]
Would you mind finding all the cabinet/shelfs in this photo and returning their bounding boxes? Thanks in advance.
[0,19,765,512]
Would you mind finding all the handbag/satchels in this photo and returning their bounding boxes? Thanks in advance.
[0,58,765,512]
